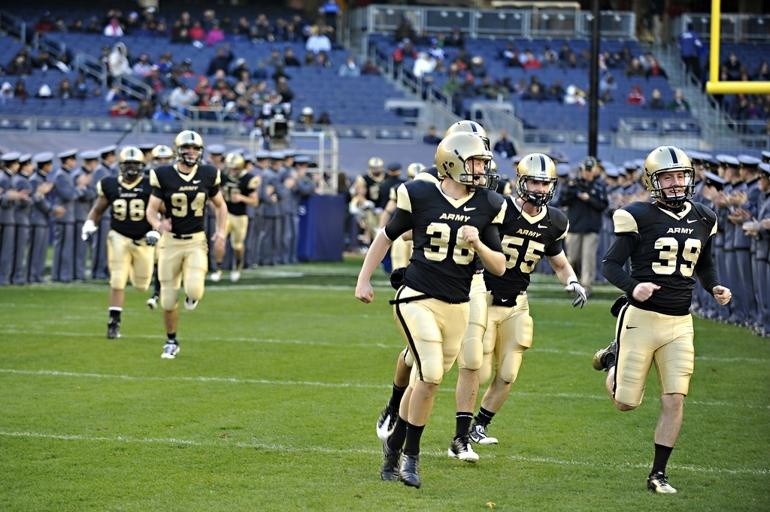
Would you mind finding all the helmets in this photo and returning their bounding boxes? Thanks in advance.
[406,162,425,177]
[515,153,559,208]
[434,119,500,191]
[367,156,384,176]
[118,148,144,182]
[151,145,173,166]
[642,146,697,209]
[174,130,205,167]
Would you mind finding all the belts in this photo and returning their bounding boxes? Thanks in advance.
[173,234,193,240]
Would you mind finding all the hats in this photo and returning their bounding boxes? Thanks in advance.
[558,158,644,179]
[140,143,153,150]
[1,145,117,165]
[208,145,310,164]
[689,150,769,192]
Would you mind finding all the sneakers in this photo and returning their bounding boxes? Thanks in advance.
[161,340,180,359]
[470,425,499,445]
[229,271,241,283]
[184,297,199,311]
[380,442,404,484]
[448,435,479,463]
[147,294,159,310]
[375,402,400,440]
[107,320,120,339]
[593,339,616,370]
[401,453,422,488]
[210,270,223,283]
[647,471,677,493]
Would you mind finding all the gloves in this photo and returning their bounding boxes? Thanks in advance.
[81,220,98,242]
[146,230,161,246]
[564,282,587,308]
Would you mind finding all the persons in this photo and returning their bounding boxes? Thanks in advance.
[470,152,587,445]
[144,129,228,361]
[593,146,733,494]
[355,132,507,489]
[376,118,508,461]
[2,146,434,286]
[1,0,336,152]
[691,149,769,336]
[341,15,769,115]
[493,134,644,283]
